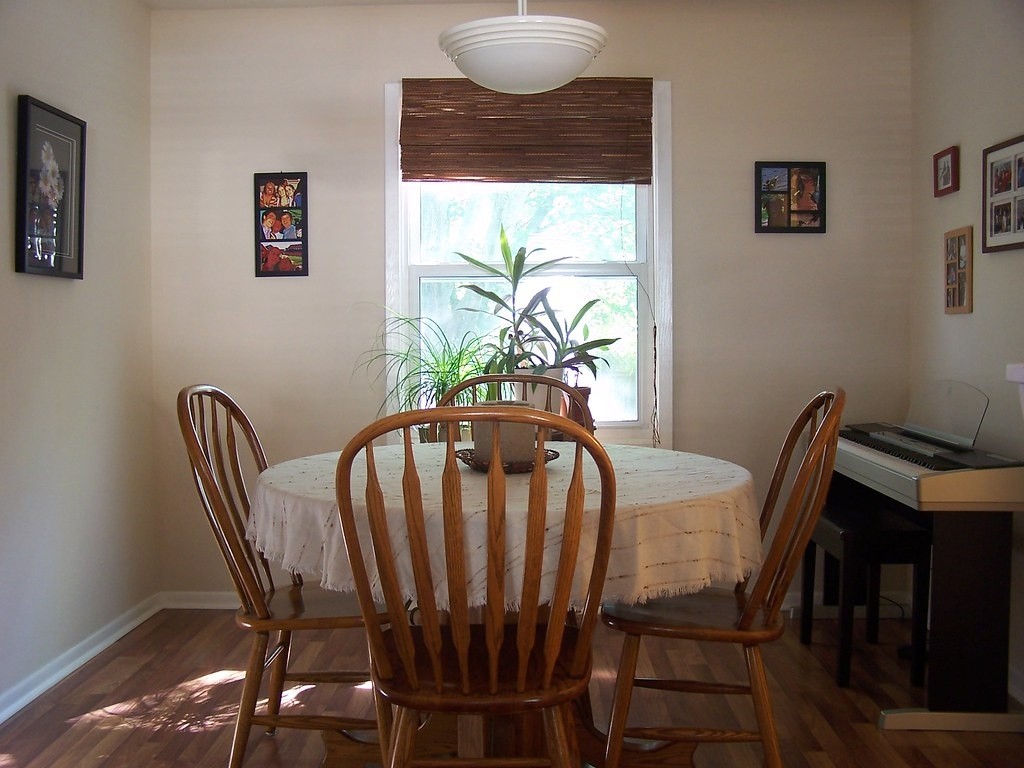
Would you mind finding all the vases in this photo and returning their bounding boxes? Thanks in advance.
[474,400,534,460]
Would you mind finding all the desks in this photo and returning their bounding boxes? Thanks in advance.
[237,442,763,768]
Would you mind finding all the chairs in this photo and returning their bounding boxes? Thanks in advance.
[333,403,618,768]
[176,384,387,768]
[600,384,846,768]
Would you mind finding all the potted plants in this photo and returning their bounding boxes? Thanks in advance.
[368,315,475,441]
[462,229,621,416]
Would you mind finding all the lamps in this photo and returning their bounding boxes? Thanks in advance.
[438,0,609,95]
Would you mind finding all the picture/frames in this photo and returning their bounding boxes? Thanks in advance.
[981,135,1024,254]
[12,95,87,280]
[252,172,308,276]
[933,146,959,197]
[752,160,827,234]
[942,225,974,314]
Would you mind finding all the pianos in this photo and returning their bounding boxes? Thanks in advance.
[799,421,1024,734]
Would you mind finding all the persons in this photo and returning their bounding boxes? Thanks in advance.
[939,161,950,186]
[993,157,1024,234]
[259,179,303,271]
[947,237,967,307]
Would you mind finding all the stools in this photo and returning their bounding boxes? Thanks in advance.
[798,499,931,690]
